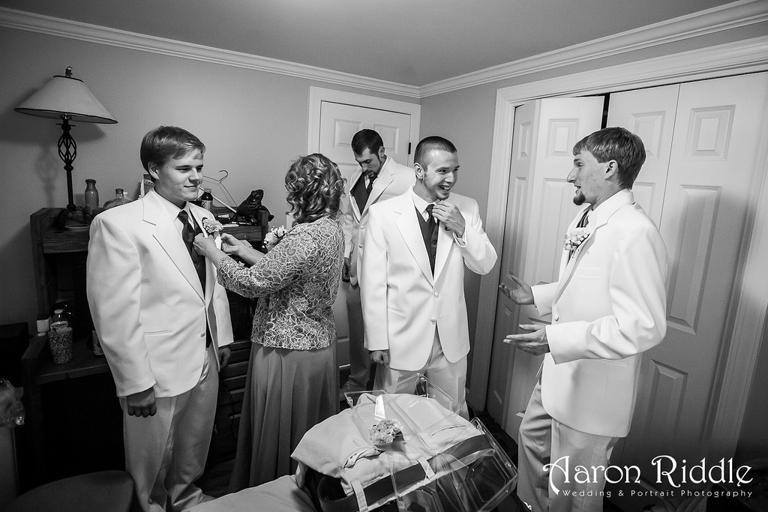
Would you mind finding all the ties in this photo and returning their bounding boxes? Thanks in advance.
[178,211,213,349]
[426,204,436,225]
[367,175,377,195]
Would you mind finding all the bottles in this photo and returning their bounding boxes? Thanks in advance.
[201,189,213,212]
[48,309,73,365]
[85,179,132,216]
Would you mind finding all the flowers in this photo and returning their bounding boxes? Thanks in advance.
[562,227,590,260]
[201,216,224,238]
[369,394,401,448]
[264,226,286,252]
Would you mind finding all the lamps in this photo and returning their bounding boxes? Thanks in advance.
[12,65,118,231]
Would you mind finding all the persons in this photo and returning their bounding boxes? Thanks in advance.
[499,128,667,512]
[189,153,345,492]
[87,125,235,512]
[340,129,420,401]
[359,135,497,422]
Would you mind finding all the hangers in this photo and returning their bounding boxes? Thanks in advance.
[198,168,238,215]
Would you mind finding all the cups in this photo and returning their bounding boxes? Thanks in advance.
[92,331,104,356]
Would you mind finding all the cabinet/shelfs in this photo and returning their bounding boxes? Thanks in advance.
[21,208,270,489]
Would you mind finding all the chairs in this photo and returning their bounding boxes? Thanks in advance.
[0,377,139,512]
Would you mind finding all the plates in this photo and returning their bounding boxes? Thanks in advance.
[64,223,89,232]
